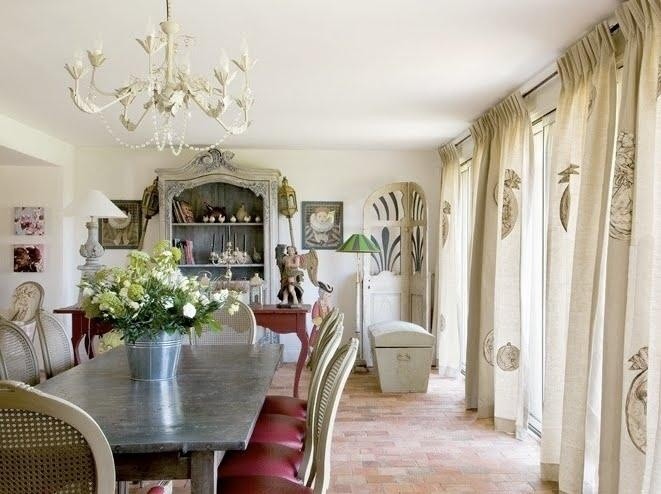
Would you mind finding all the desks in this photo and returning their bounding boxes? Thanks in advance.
[54,303,311,398]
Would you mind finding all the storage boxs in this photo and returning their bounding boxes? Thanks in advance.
[368,321,435,393]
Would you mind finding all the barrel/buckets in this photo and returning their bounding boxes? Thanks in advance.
[129,382,183,433]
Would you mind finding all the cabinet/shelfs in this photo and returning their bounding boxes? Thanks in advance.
[172,222,263,267]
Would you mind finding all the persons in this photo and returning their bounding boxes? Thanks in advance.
[279,245,300,306]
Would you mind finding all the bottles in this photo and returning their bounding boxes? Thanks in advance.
[236,202,247,221]
[251,272,264,304]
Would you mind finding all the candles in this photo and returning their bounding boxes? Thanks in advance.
[209,225,247,252]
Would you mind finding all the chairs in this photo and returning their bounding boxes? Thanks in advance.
[0,380,116,494]
[248,312,344,450]
[217,338,359,494]
[261,307,340,419]
[215,327,343,485]
[189,295,256,345]
[35,309,74,380]
[0,319,40,387]
[0,281,45,343]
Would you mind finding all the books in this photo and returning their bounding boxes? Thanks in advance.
[172,198,194,223]
[174,237,195,265]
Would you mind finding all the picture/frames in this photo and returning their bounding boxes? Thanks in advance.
[301,202,343,250]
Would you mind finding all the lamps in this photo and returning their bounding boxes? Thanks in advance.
[336,235,382,373]
[63,0,255,156]
[59,189,129,309]
[279,177,297,247]
[138,177,159,251]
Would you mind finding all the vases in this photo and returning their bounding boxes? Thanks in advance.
[98,200,142,250]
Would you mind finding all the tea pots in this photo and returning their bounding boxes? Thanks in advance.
[204,203,227,221]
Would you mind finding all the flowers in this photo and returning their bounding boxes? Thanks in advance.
[77,240,243,354]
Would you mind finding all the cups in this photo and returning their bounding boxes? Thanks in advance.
[210,280,250,290]
